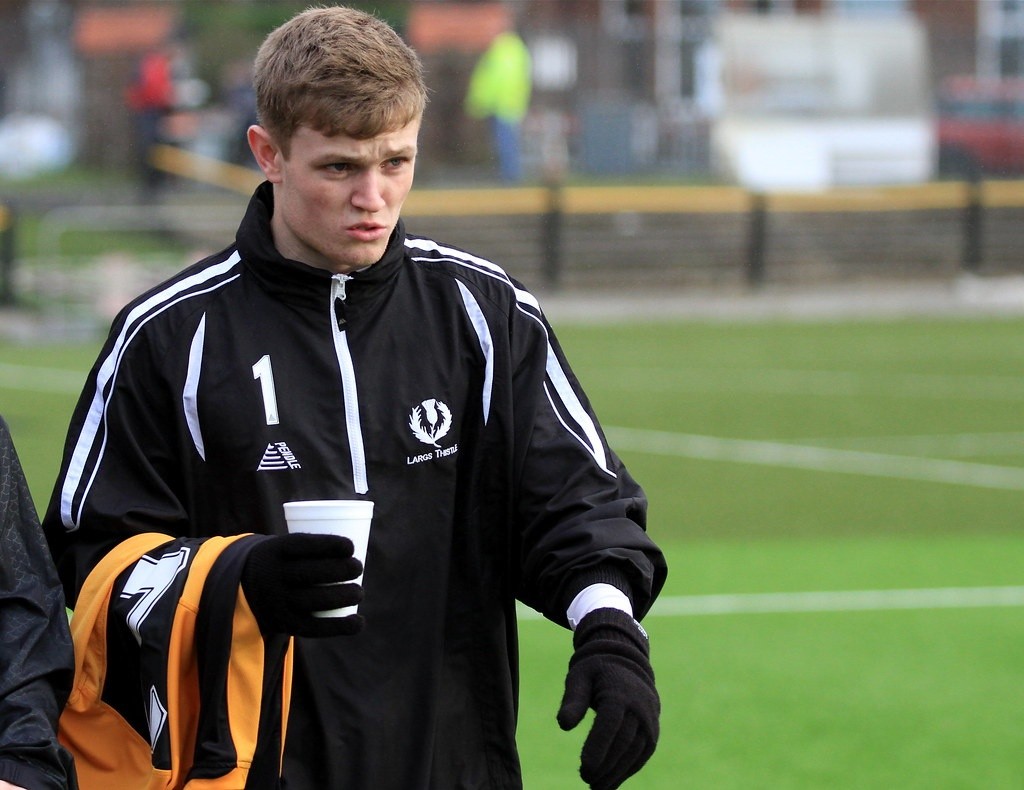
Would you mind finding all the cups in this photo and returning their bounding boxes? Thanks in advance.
[283,498,375,619]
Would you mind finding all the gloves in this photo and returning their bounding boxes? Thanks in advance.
[556,607,661,790]
[241,533,368,639]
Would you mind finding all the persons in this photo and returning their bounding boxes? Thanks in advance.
[463,6,532,187]
[42,8,668,790]
[521,9,583,184]
[119,19,204,201]
[0,415,78,790]
